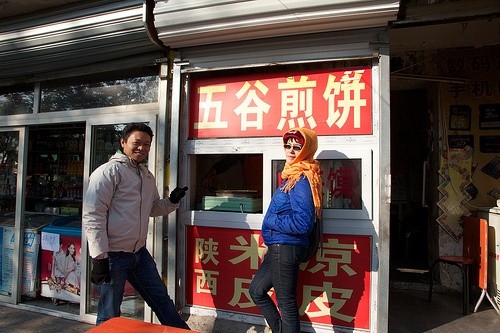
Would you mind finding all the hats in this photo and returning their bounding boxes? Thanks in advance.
[283,128,305,144]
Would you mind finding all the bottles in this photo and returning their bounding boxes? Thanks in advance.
[52,184,83,199]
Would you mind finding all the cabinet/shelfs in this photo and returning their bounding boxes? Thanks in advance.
[0,123,151,222]
[0,124,148,216]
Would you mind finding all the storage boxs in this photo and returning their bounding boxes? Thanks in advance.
[45,207,79,216]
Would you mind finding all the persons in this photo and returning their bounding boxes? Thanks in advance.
[248,127,322,333]
[81,122,191,330]
[50,237,81,289]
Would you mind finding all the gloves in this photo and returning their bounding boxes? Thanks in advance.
[169,186,188,204]
[91,257,111,285]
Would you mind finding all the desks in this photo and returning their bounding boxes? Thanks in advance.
[391,200,417,242]
[85,317,203,333]
[463,218,500,315]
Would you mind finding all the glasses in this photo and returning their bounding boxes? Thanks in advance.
[283,145,302,151]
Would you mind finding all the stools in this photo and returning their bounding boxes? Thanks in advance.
[429,256,474,315]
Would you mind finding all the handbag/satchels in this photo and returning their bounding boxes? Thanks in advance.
[302,218,323,262]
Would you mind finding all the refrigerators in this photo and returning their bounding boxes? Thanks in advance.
[38,215,143,318]
[0,210,64,300]
[476,207,500,309]
